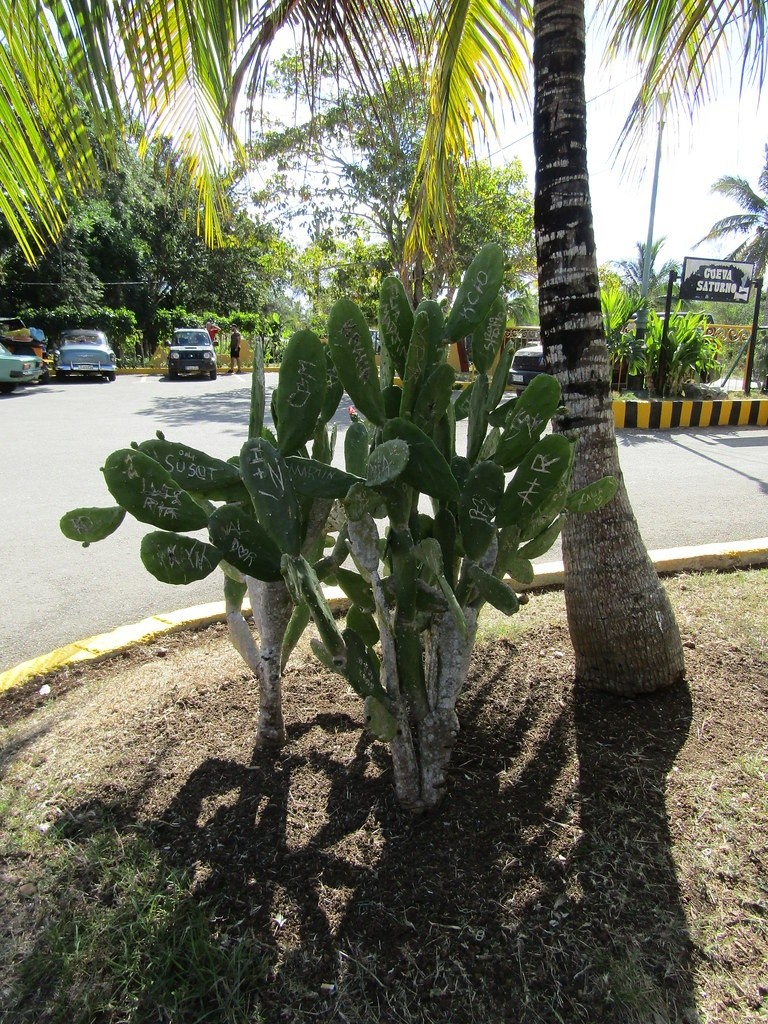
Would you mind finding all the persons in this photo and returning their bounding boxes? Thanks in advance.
[227,324,241,373]
[205,321,222,342]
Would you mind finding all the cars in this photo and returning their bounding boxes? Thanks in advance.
[369,329,381,355]
[0,342,48,395]
[53,329,117,382]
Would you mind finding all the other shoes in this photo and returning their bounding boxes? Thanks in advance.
[236,370,241,374]
[227,370,234,373]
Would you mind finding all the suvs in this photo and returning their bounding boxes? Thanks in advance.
[165,329,219,380]
[506,311,721,398]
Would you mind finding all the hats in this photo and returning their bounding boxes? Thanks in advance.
[230,324,237,328]
